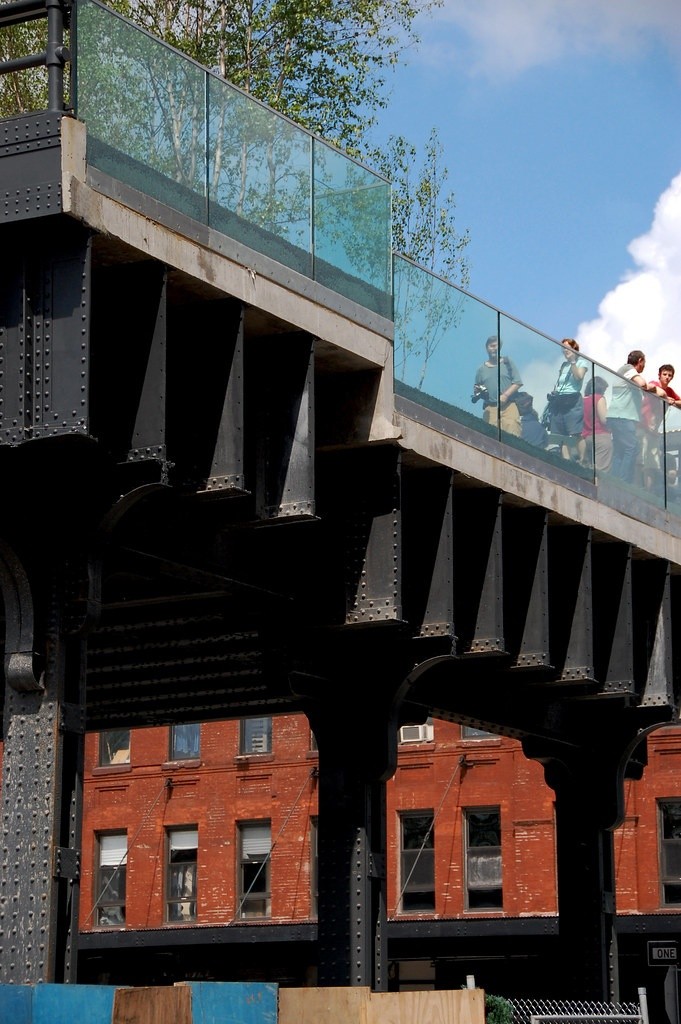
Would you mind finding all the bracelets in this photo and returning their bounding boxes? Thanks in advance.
[570,362,576,365]
[504,393,509,398]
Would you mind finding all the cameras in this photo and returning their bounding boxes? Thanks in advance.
[547,390,560,402]
[470,383,490,404]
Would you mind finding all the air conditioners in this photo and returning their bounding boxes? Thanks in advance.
[399,724,429,745]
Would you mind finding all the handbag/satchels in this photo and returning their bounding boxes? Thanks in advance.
[514,392,533,415]
[548,392,580,411]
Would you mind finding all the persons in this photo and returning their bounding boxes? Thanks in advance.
[550,338,588,464]
[606,350,646,483]
[521,409,548,449]
[474,335,523,437]
[581,376,613,473]
[388,960,400,992]
[633,365,681,503]
